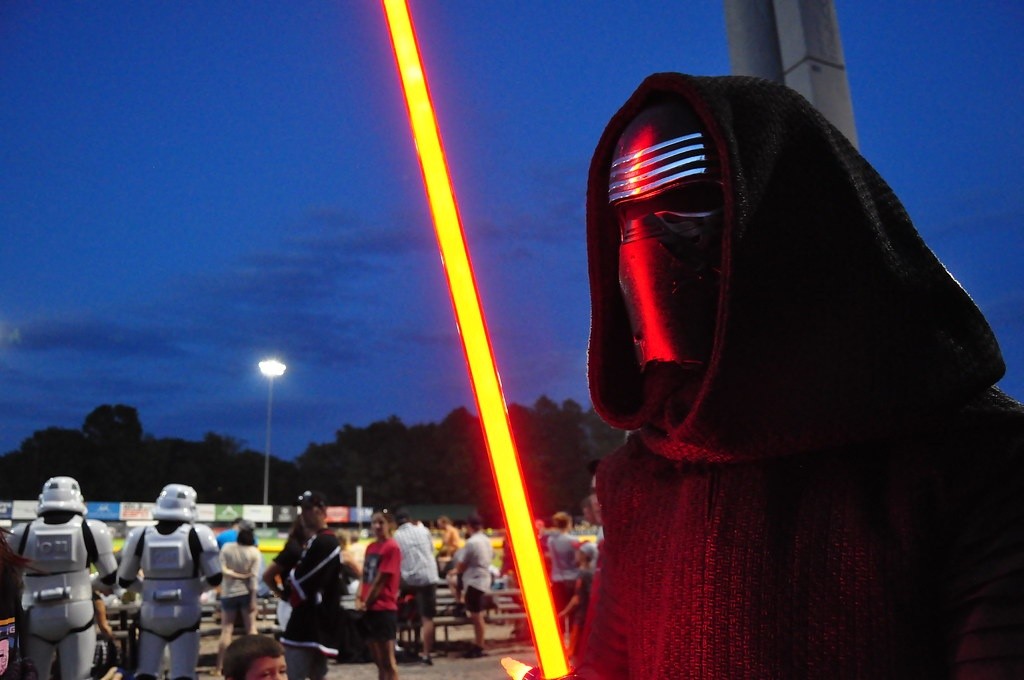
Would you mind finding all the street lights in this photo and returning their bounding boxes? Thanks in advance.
[257,356,287,527]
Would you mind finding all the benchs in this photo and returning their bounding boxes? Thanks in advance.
[398,587,528,660]
[96,590,283,670]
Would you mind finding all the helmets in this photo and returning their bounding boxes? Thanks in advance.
[37,476,88,516]
[152,484,197,522]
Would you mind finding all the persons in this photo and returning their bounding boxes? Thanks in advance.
[521,70,1023,679]
[209,518,259,677]
[535,511,602,633]
[222,635,288,680]
[262,498,327,652]
[8,476,119,680]
[0,526,38,680]
[55,591,113,679]
[387,512,440,664]
[434,516,464,578]
[215,517,259,562]
[445,515,494,658]
[281,506,363,680]
[115,483,223,680]
[356,508,402,680]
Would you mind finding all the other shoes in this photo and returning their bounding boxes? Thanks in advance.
[463,646,488,658]
[452,603,466,617]
[417,656,433,665]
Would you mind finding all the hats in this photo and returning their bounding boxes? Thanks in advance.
[239,520,256,529]
